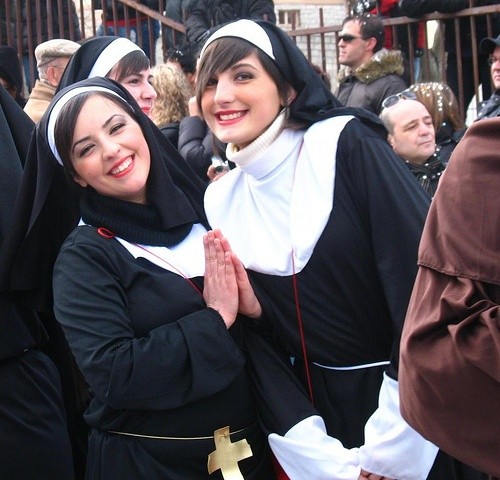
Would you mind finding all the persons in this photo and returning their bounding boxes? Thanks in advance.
[196,19,491,480]
[0,0,500,480]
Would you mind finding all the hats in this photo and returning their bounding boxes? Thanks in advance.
[34,39,81,67]
[53,35,146,97]
[200,18,329,124]
[23,76,152,226]
[0,47,22,92]
[479,35,500,54]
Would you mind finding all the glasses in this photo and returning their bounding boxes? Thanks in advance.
[337,34,363,43]
[380,88,417,111]
[487,57,500,65]
